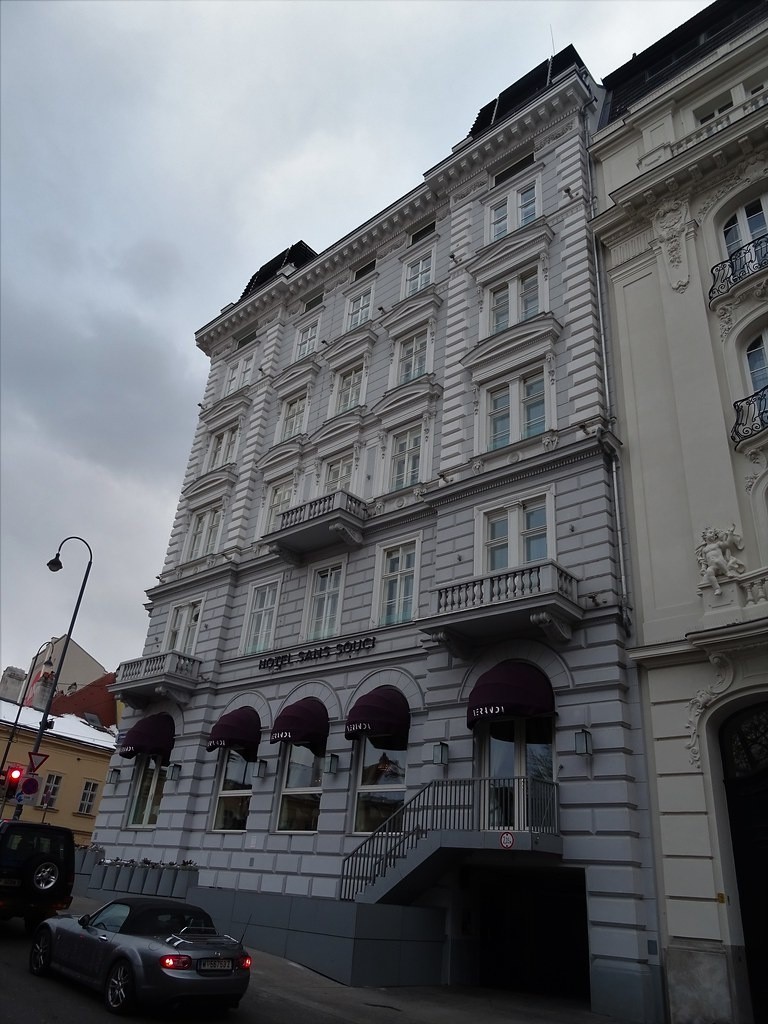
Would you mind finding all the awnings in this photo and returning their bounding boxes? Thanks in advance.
[467,660,556,728]
[120,713,174,760]
[270,700,328,747]
[207,707,261,752]
[345,687,411,739]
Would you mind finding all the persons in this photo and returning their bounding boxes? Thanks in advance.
[699,526,735,595]
[81,918,86,925]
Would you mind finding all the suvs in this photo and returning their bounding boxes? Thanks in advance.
[0,818,75,932]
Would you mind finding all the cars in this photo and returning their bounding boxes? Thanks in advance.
[29,899,252,1016]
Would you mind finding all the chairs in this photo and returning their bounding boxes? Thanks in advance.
[168,913,185,923]
[17,839,35,852]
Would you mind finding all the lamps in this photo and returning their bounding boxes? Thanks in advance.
[433,742,449,766]
[252,759,268,777]
[165,764,182,780]
[323,753,339,774]
[106,769,121,783]
[575,729,593,757]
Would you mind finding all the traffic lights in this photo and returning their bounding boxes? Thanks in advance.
[10,768,23,781]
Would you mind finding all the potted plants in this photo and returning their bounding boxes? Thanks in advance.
[114,858,138,893]
[142,859,166,897]
[80,843,105,875]
[172,859,201,898]
[155,861,180,897]
[101,857,125,890]
[74,844,88,874]
[128,858,152,894]
[87,859,108,889]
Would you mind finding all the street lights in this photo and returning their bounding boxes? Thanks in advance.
[13,536,93,821]
[0,641,54,779]
